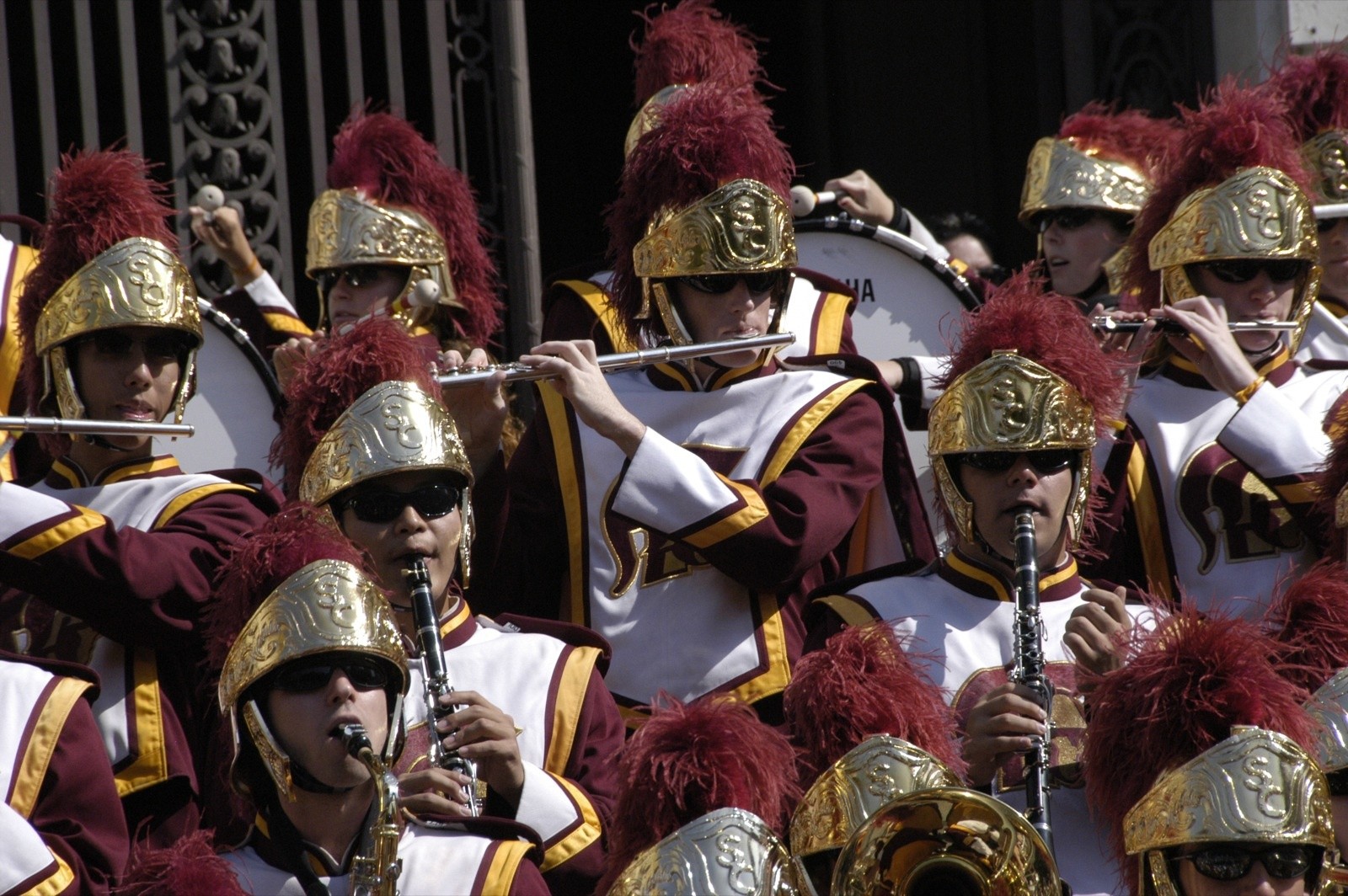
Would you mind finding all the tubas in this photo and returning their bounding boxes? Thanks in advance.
[830,785,1063,895]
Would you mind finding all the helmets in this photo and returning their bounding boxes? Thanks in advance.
[260,295,477,589]
[592,689,819,896]
[1258,28,1348,218]
[1017,98,1186,222]
[17,134,204,458]
[1231,554,1348,774]
[195,501,411,824]
[783,614,987,896]
[1070,575,1347,896]
[928,258,1163,565]
[1113,68,1322,360]
[602,0,815,374]
[304,96,509,349]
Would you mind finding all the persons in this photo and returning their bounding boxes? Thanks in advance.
[592,574,1347,896]
[0,649,130,896]
[828,45,1348,642]
[435,54,885,746]
[799,254,1199,896]
[0,108,534,834]
[271,304,627,896]
[200,500,554,896]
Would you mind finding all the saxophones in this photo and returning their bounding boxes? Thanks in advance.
[326,720,399,896]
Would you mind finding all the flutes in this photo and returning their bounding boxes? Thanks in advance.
[399,554,483,818]
[1084,315,1304,334]
[972,510,1075,896]
[1,414,196,441]
[432,330,796,390]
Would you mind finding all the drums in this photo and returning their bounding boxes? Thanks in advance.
[149,297,294,497]
[794,212,989,574]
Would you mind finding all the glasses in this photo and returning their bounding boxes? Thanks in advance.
[314,264,397,291]
[954,448,1080,475]
[674,270,781,295]
[1168,843,1319,882]
[337,484,462,524]
[1197,259,1309,284]
[74,329,189,360]
[1316,217,1348,231]
[1326,768,1348,796]
[1030,208,1097,232]
[271,651,392,693]
